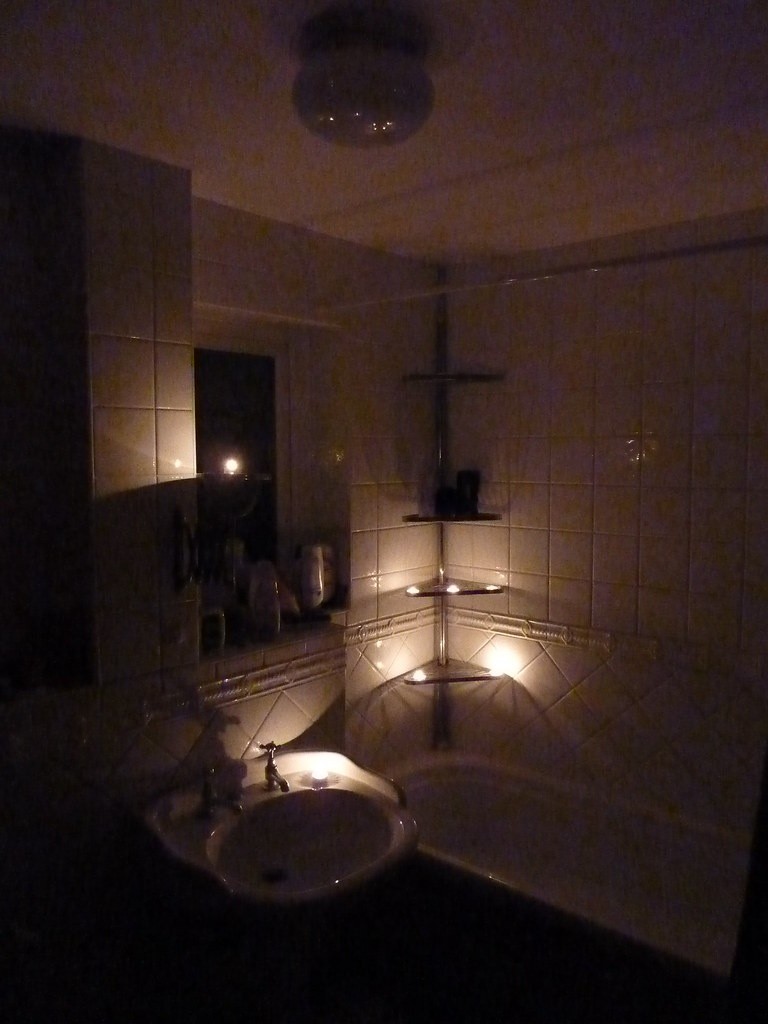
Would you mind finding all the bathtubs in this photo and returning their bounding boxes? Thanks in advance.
[380,748,752,978]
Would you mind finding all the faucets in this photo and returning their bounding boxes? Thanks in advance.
[258,743,290,793]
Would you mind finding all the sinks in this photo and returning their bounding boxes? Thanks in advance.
[145,750,417,908]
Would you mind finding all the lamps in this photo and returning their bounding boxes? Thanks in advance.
[289,0,434,153]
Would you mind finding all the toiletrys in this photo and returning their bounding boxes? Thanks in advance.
[150,506,332,664]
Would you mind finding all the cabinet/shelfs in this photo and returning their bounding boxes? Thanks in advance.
[405,374,506,684]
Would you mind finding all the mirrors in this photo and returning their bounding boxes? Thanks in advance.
[194,349,277,645]
